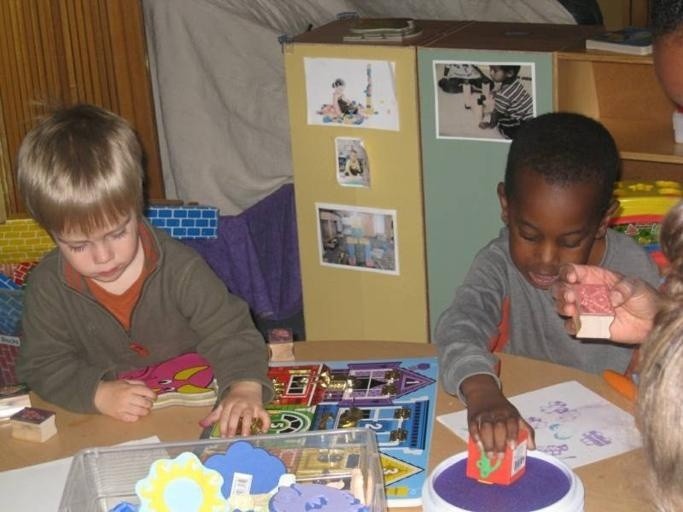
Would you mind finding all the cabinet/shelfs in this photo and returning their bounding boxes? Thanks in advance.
[555,26,683,187]
[416,22,592,345]
[284,19,469,347]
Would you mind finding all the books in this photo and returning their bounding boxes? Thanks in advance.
[581,25,661,57]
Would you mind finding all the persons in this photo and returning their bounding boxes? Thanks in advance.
[551,1,683,510]
[437,64,494,110]
[342,146,364,178]
[14,101,276,440]
[475,66,533,140]
[434,113,661,452]
[328,77,364,115]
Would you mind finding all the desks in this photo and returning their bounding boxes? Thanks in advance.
[0,341,659,512]
